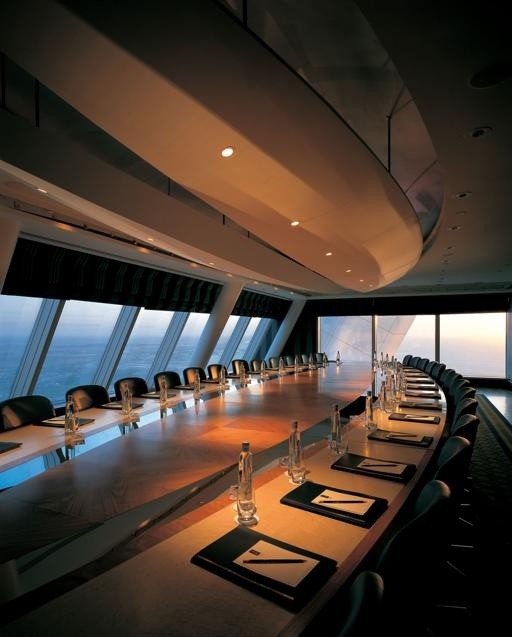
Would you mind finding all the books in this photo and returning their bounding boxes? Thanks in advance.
[248,371,261,374]
[173,384,205,390]
[398,401,442,410]
[301,363,309,365]
[407,384,439,390]
[1,441,22,452]
[404,372,428,377]
[388,413,440,424]
[190,525,337,610]
[34,416,95,427]
[98,400,144,410]
[402,369,422,372]
[401,365,414,369]
[406,378,434,383]
[332,453,415,481]
[265,367,279,370]
[280,481,388,527]
[202,378,229,383]
[328,360,336,362]
[367,428,433,446]
[316,362,323,364]
[284,365,295,367]
[225,374,249,378]
[405,391,441,398]
[140,391,176,399]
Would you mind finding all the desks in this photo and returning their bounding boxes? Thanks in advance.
[0,363,447,637]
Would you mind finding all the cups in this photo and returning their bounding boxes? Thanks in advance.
[334,427,351,458]
[235,484,258,527]
[289,451,307,485]
[367,409,379,438]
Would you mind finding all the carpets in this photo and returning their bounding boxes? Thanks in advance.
[384,393,512,637]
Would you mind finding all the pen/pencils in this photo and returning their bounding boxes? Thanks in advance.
[242,558,306,563]
[48,418,67,420]
[318,500,363,502]
[390,433,417,436]
[408,415,429,417]
[361,464,398,466]
[416,402,432,404]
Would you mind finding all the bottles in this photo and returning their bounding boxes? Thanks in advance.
[288,420,303,476]
[391,356,395,369]
[279,356,284,372]
[323,367,327,378]
[385,354,389,368]
[193,369,201,392]
[279,375,284,386]
[380,381,388,410]
[309,370,314,379]
[380,367,383,377]
[331,404,342,443]
[241,384,247,388]
[63,395,76,433]
[374,351,377,363]
[336,351,340,365]
[261,360,266,377]
[218,391,224,397]
[373,363,377,374]
[160,408,167,430]
[219,365,225,386]
[65,445,75,463]
[124,421,134,435]
[121,382,130,414]
[159,376,168,401]
[337,366,341,376]
[295,355,299,370]
[261,379,268,384]
[380,352,383,365]
[239,361,245,381]
[238,441,254,500]
[323,352,327,366]
[195,398,200,417]
[309,354,313,368]
[295,373,299,382]
[365,391,374,426]
[390,375,396,398]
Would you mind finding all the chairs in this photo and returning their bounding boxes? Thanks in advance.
[208,364,228,379]
[425,362,437,373]
[449,378,470,395]
[432,435,474,577]
[302,354,308,362]
[286,356,293,365]
[410,356,420,366]
[451,413,481,526]
[417,358,429,368]
[232,359,249,373]
[453,386,476,404]
[66,385,110,412]
[450,397,478,492]
[316,352,323,361]
[183,367,206,385]
[154,371,182,390]
[0,395,56,432]
[404,355,412,364]
[338,570,385,636]
[440,369,455,386]
[269,357,281,367]
[114,377,149,400]
[432,363,446,378]
[376,480,467,636]
[251,359,267,370]
[445,372,461,388]
